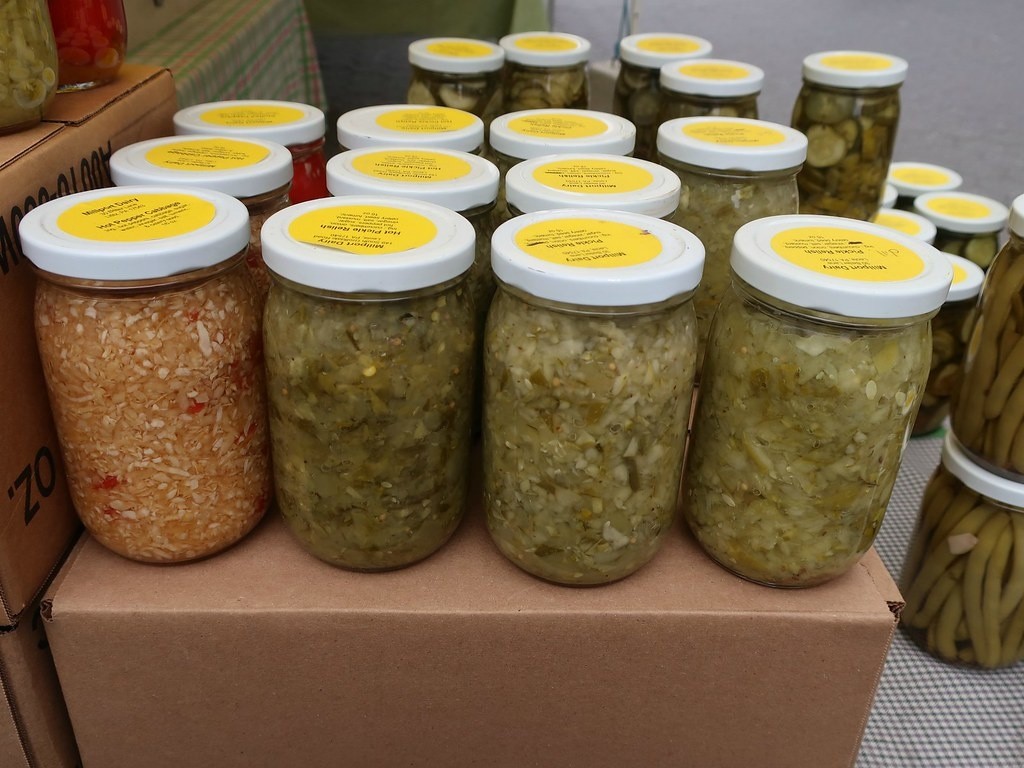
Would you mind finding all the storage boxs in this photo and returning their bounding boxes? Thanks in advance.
[0,60,182,634]
[39,481,908,768]
[0,601,79,767]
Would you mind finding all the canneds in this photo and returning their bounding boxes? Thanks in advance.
[898,191,1024,674]
[18,31,1011,589]
[0,0,127,133]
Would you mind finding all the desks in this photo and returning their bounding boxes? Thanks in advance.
[123,0,329,113]
